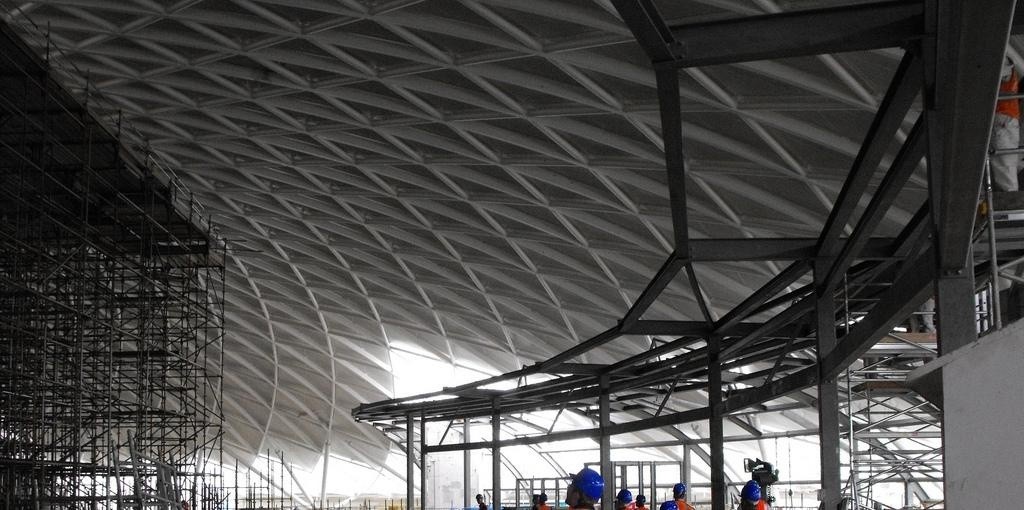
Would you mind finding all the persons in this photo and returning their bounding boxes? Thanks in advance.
[476,494,488,510]
[531,493,550,510]
[614,484,695,510]
[990,56,1020,192]
[565,468,606,510]
[736,481,772,510]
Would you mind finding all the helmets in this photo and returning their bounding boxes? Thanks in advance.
[616,489,632,502]
[569,468,606,501]
[659,500,677,510]
[636,495,646,502]
[476,494,484,499]
[738,480,762,501]
[673,483,686,493]
